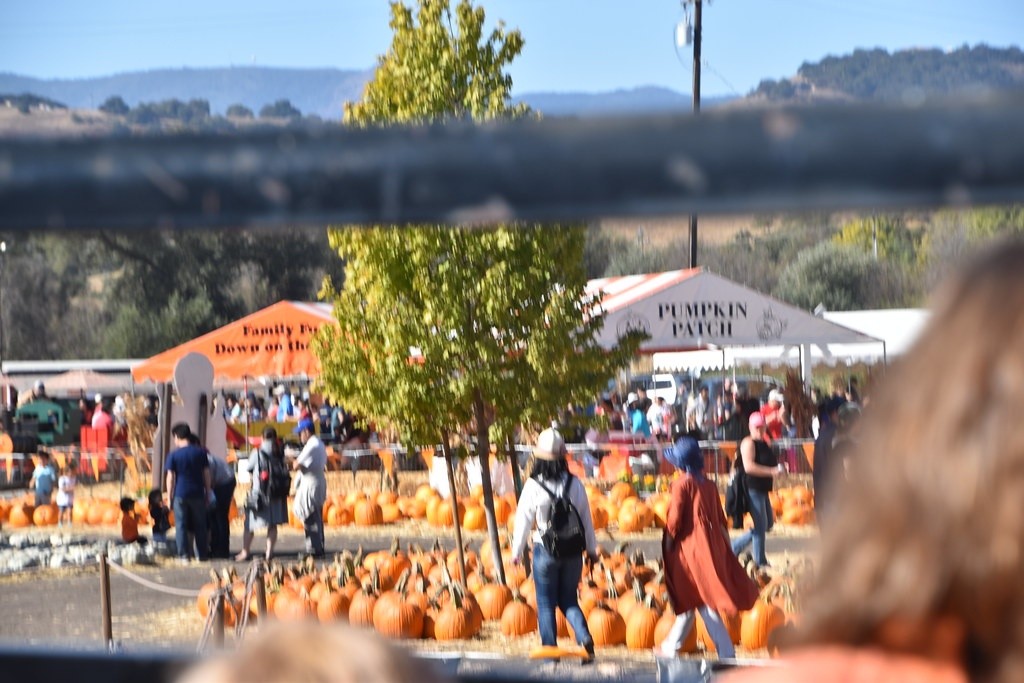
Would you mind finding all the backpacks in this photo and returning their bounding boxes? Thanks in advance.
[530,471,586,557]
[254,445,291,496]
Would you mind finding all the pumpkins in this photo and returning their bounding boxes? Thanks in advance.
[0,479,816,659]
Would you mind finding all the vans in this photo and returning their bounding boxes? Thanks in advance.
[627,372,786,412]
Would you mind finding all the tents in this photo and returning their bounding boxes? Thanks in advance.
[0,266,928,411]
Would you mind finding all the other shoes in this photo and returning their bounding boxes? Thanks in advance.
[579,634,596,665]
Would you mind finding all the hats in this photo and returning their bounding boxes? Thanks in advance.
[273,384,285,394]
[663,436,704,483]
[749,412,764,428]
[768,389,785,401]
[627,391,638,402]
[293,418,313,433]
[531,427,566,461]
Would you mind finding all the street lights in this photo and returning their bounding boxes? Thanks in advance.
[706,342,726,442]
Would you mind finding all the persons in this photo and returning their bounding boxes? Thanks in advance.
[165,421,236,566]
[291,421,327,560]
[0,370,879,549]
[120,487,172,563]
[715,229,1024,683]
[235,426,292,563]
[725,412,781,572]
[660,439,759,672]
[511,427,596,673]
